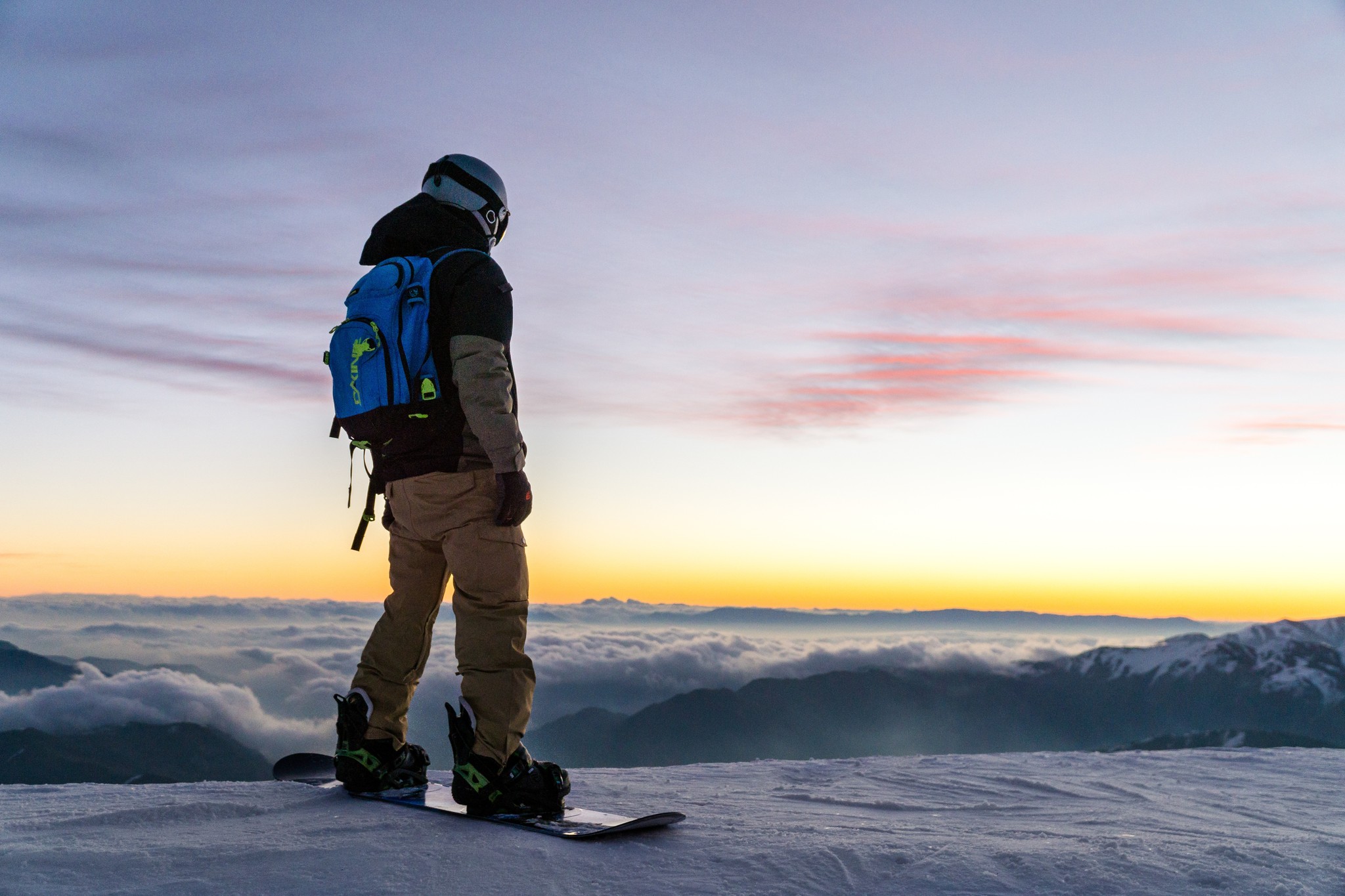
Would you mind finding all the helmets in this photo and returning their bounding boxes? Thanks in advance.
[422,154,508,252]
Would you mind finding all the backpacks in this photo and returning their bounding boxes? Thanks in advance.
[324,249,492,454]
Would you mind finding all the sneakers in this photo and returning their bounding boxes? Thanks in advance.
[333,687,431,790]
[444,697,571,806]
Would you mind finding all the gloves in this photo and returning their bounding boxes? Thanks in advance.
[495,469,532,528]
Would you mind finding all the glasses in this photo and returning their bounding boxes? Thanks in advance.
[493,221,510,245]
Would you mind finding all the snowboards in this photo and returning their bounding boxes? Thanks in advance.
[272,752,687,838]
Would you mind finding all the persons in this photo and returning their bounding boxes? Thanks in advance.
[333,154,573,813]
